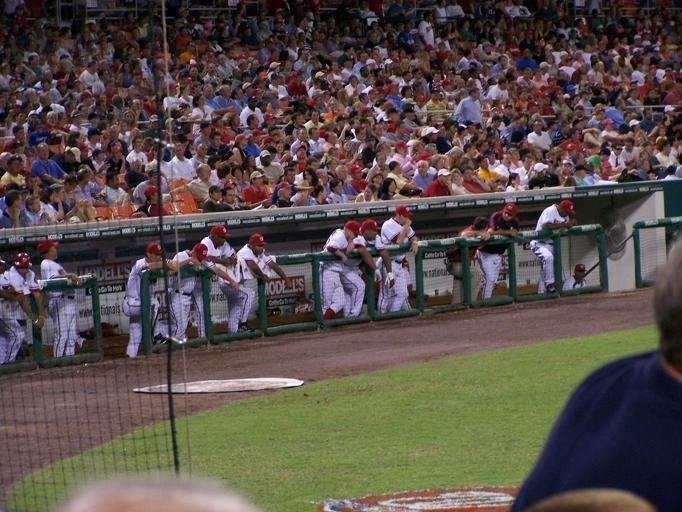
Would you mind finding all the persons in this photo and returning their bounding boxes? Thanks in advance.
[0,0,682,365]
[511,235,681,511]
[526,488,651,512]
[54,465,259,511]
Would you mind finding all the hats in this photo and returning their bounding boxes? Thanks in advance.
[144,186,157,196]
[664,106,675,112]
[314,71,324,78]
[422,127,439,137]
[235,112,276,145]
[560,200,575,213]
[270,62,281,70]
[393,141,409,148]
[147,243,163,254]
[438,168,451,177]
[85,112,105,157]
[150,114,158,122]
[37,241,58,252]
[629,119,640,127]
[534,163,549,173]
[504,203,516,215]
[349,164,362,173]
[212,224,232,238]
[575,264,586,271]
[277,180,313,189]
[396,204,413,217]
[250,233,268,246]
[539,62,552,68]
[345,219,381,234]
[71,147,82,163]
[192,243,209,259]
[250,150,271,179]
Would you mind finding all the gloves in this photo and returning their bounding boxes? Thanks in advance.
[385,272,394,288]
[374,268,382,282]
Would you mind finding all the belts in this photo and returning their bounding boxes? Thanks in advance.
[175,290,193,296]
[62,295,74,299]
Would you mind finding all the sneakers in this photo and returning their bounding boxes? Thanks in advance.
[152,335,168,346]
[547,285,557,293]
[236,326,252,332]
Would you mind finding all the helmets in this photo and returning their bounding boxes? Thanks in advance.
[14,252,31,269]
